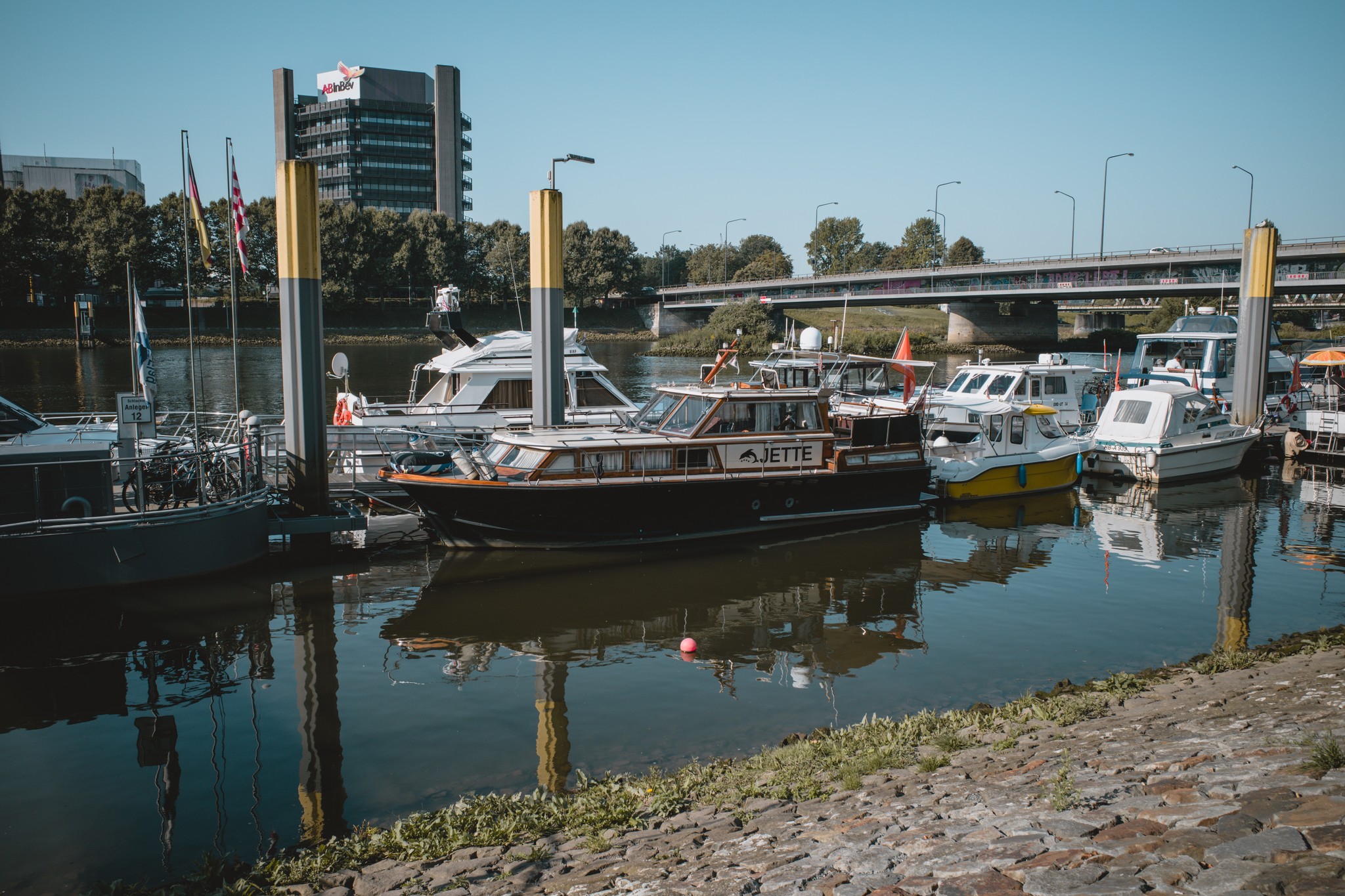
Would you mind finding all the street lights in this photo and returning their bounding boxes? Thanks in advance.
[1055,190,1075,259]
[725,218,747,286]
[926,209,945,267]
[1099,153,1134,256]
[759,234,775,280]
[662,230,682,289]
[932,181,961,268]
[690,244,710,285]
[827,223,846,275]
[1232,165,1254,230]
[814,202,838,279]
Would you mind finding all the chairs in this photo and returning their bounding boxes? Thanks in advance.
[1311,384,1345,401]
[1079,394,1098,423]
[1154,359,1165,367]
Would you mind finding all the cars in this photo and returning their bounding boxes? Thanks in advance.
[607,292,631,296]
[1149,247,1176,253]
[862,269,881,272]
[641,287,654,293]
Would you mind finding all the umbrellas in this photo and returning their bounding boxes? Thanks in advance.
[1299,349,1345,410]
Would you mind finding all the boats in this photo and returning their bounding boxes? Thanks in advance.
[696,272,1345,514]
[226,243,671,487]
[370,292,941,552]
[0,396,281,594]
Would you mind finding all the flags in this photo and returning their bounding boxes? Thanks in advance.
[986,386,990,399]
[728,355,740,375]
[889,327,916,405]
[1221,401,1228,414]
[715,354,727,370]
[231,155,250,284]
[1191,368,1200,391]
[791,320,797,345]
[188,148,212,271]
[1264,401,1268,415]
[1286,353,1301,409]
[1010,389,1014,404]
[134,277,157,408]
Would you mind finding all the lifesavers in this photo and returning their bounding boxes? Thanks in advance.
[332,397,347,425]
[1280,394,1297,413]
[243,438,250,472]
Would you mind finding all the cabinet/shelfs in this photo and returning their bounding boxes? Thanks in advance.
[833,414,871,438]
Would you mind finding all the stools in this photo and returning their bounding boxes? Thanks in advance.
[1265,411,1279,427]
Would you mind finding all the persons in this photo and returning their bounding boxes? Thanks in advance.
[1287,352,1295,366]
[1164,355,1183,369]
[1329,370,1345,394]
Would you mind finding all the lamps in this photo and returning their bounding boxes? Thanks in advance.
[978,349,983,355]
[736,329,742,337]
[734,382,739,390]
[723,343,728,349]
